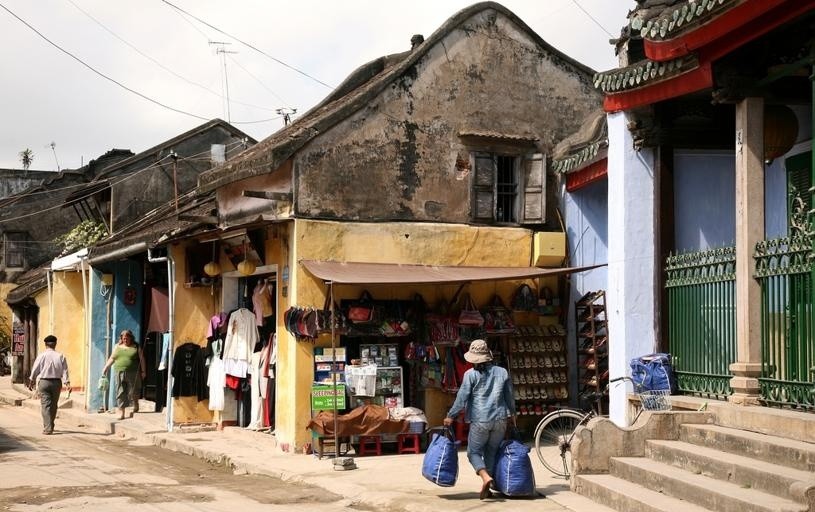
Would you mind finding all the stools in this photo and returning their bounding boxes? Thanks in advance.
[358,434,421,457]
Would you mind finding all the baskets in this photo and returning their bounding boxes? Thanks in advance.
[638,389,672,412]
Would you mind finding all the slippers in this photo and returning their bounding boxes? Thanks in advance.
[515,403,561,416]
[509,324,568,400]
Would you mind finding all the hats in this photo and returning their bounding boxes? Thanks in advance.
[463,339,494,364]
[284,307,319,345]
[45,335,57,342]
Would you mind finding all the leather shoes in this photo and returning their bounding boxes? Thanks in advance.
[480,478,494,500]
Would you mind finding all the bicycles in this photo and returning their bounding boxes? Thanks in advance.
[534,371,672,480]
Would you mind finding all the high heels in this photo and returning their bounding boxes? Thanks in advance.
[576,290,608,402]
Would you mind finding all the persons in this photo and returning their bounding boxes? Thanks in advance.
[27,334,73,435]
[101,329,147,421]
[443,337,518,500]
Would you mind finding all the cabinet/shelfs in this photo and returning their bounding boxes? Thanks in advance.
[349,365,405,445]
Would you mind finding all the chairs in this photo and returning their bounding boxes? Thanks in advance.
[309,392,350,460]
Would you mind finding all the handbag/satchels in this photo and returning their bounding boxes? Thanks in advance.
[422,424,461,488]
[404,293,458,391]
[490,424,536,497]
[457,293,513,335]
[344,290,411,338]
[533,286,562,317]
[510,283,537,310]
[314,284,345,335]
[628,352,676,394]
